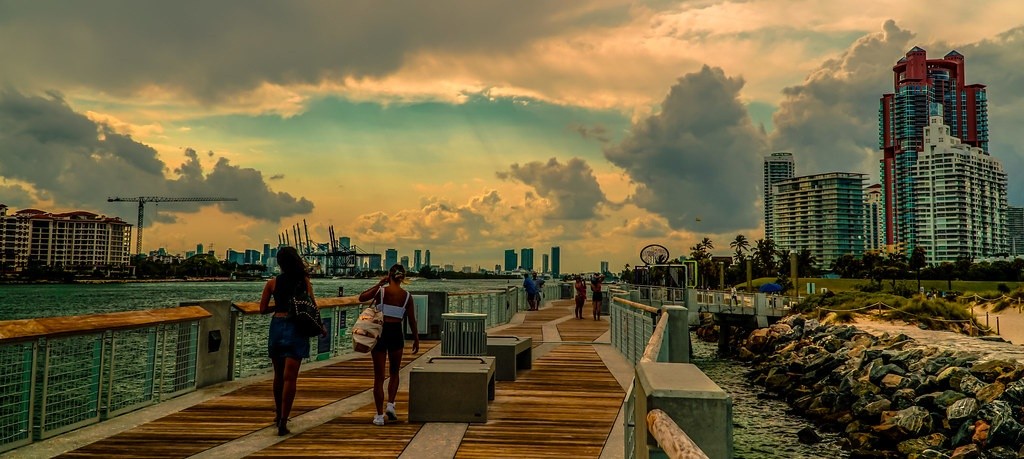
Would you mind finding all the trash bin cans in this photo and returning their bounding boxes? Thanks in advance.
[560,283,575,299]
[600,285,611,314]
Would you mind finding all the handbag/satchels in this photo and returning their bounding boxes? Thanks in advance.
[351,287,385,354]
[290,277,323,338]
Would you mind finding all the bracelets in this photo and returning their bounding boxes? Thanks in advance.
[320,322,324,326]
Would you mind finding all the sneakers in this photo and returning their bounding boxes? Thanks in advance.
[385,402,397,420]
[373,415,384,425]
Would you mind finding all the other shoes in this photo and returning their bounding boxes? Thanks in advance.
[273,408,290,436]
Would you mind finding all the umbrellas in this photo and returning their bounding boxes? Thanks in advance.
[760,283,783,292]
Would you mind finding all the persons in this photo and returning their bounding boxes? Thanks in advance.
[730,283,739,306]
[573,273,606,321]
[359,264,420,425]
[523,272,546,311]
[260,246,328,436]
